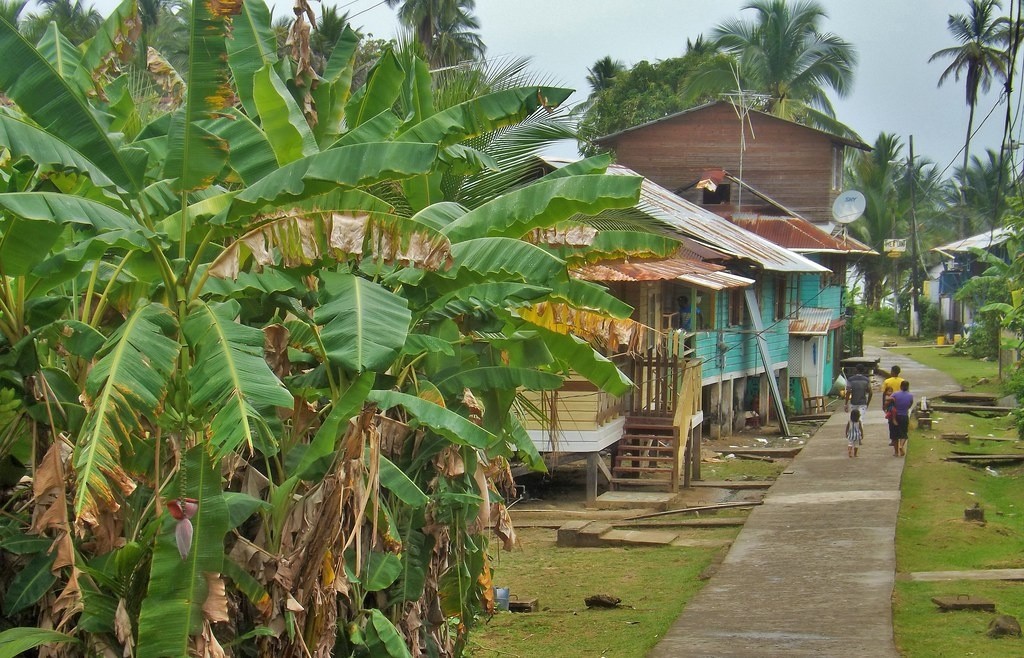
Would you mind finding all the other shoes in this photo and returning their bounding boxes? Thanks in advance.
[893,454,898,457]
[892,419,898,427]
[889,443,894,446]
[900,446,905,456]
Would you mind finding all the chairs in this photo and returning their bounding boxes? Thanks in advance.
[800,376,826,415]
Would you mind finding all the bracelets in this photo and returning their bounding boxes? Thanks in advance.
[844,405,848,406]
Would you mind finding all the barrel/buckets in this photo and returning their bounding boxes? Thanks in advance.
[937,336,944,346]
[954,334,961,345]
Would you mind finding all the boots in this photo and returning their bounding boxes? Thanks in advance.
[854,447,859,457]
[848,446,853,457]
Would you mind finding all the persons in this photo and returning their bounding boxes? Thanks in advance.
[846,409,864,458]
[844,364,873,445]
[677,296,704,357]
[880,365,914,457]
